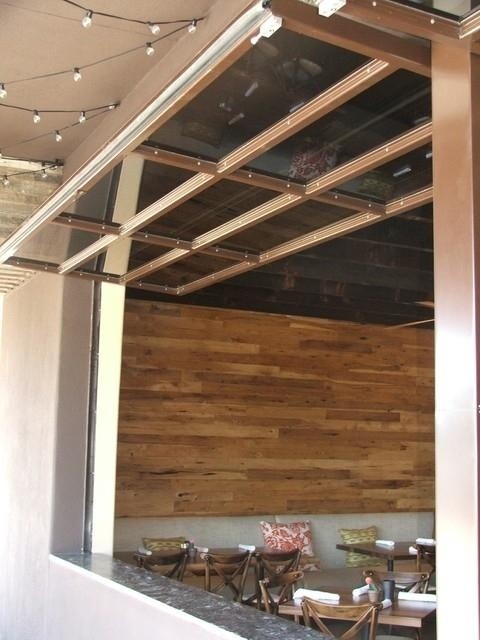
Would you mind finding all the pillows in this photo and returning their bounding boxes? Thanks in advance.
[337,525,386,569]
[142,536,188,554]
[258,519,322,575]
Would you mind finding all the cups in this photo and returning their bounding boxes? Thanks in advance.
[184,538,197,558]
[368,591,381,602]
[383,580,394,602]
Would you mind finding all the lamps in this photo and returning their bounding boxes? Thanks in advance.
[0,0,206,186]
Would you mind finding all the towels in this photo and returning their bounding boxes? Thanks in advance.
[375,539,394,546]
[416,538,435,546]
